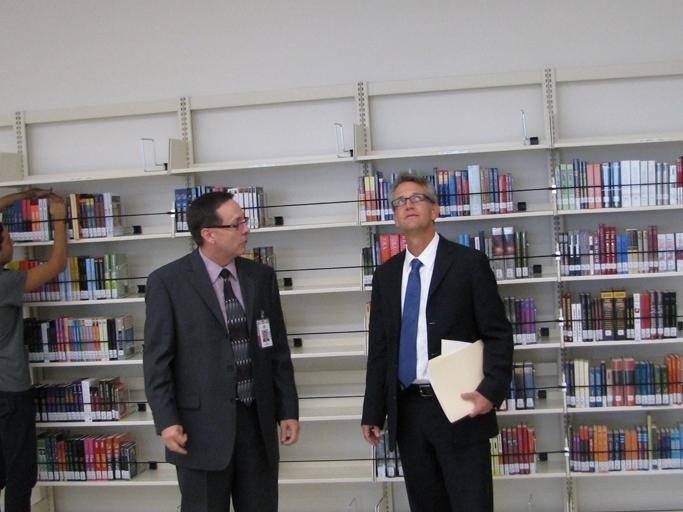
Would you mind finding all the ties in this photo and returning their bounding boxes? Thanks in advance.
[220,269,255,405]
[397,259,424,387]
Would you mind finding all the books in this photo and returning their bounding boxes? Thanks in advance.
[498,360,535,410]
[375,430,403,477]
[558,223,683,277]
[503,296,537,346]
[358,164,529,280]
[173,186,277,275]
[568,413,683,473]
[488,422,537,475]
[564,353,683,408]
[556,158,683,211]
[0,192,137,481]
[561,287,678,342]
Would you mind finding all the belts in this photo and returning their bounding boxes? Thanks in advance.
[396,383,435,399]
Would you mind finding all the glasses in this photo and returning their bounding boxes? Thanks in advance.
[391,192,436,208]
[206,217,250,229]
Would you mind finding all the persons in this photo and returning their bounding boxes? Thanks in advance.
[0,188,66,511]
[359,175,514,511]
[142,192,298,512]
